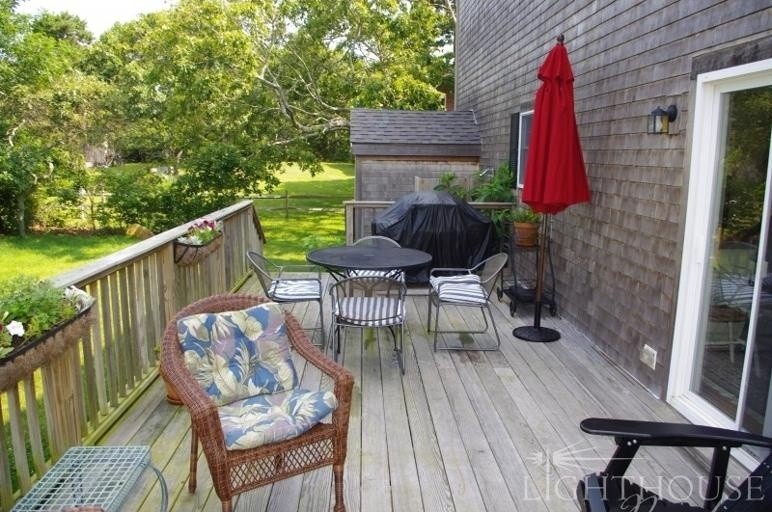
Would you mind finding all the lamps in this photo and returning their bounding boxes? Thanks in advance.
[647,104,677,134]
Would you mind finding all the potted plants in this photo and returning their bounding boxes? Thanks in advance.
[511,206,543,246]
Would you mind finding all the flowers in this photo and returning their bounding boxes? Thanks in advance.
[176,219,221,246]
[1,273,87,359]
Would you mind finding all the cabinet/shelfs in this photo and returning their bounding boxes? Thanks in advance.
[497,216,557,317]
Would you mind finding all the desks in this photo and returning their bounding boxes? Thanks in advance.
[12,446,169,511]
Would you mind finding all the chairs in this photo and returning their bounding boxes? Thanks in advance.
[160,292,354,512]
[577,414,772,511]
[704,240,772,378]
[427,253,508,353]
[325,276,407,374]
[341,235,407,299]
[246,249,326,350]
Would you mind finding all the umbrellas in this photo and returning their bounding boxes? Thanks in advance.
[521,34,590,328]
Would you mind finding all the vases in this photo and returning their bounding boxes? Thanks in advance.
[0,286,97,396]
[174,230,226,267]
